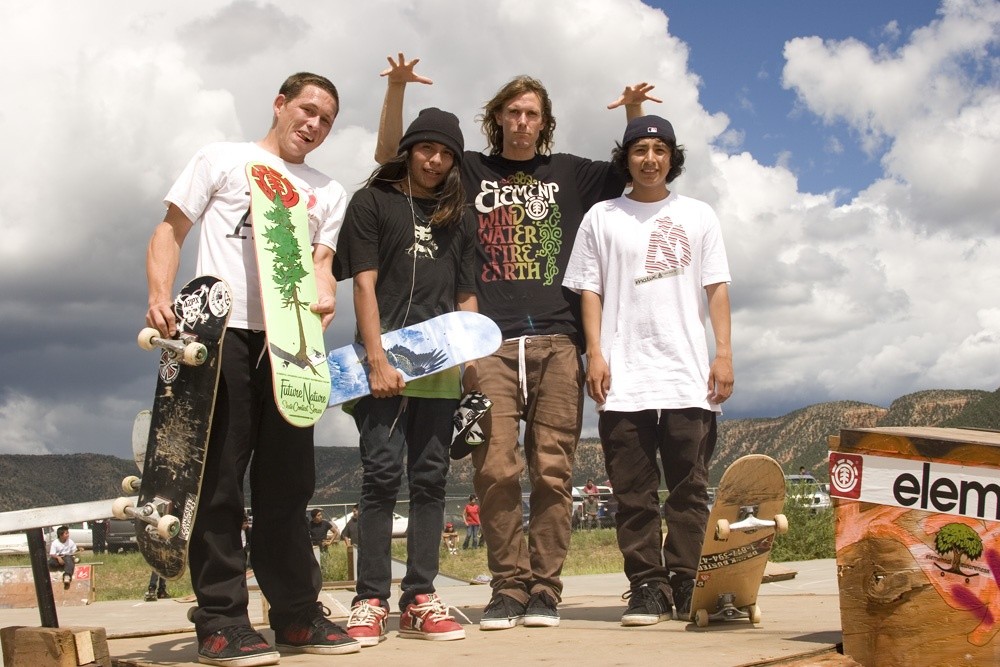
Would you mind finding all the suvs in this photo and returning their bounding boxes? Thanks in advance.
[785,474,831,512]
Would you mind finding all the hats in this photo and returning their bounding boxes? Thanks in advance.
[446,523,454,528]
[622,115,675,146]
[396,107,464,162]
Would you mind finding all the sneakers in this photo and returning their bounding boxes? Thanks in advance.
[479,591,526,629]
[669,573,698,620]
[399,590,473,641]
[197,621,279,666]
[620,584,673,624]
[523,589,559,626]
[273,603,361,656]
[346,595,391,646]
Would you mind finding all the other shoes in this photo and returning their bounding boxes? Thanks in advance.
[147,589,157,601]
[159,590,173,598]
[65,576,70,583]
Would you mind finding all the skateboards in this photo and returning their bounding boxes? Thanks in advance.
[245,160,330,428]
[113,274,233,581]
[690,452,787,626]
[326,310,504,408]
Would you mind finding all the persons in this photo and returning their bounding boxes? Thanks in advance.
[309,509,338,570]
[332,105,485,647]
[798,465,813,488]
[559,116,735,627]
[373,51,662,630]
[47,526,80,585]
[342,504,359,581]
[92,519,107,555]
[442,522,460,555]
[145,571,171,601]
[240,516,252,571]
[572,505,583,530]
[583,495,601,531]
[462,495,481,551]
[145,74,360,667]
[582,479,600,499]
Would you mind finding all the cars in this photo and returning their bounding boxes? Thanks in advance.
[306,506,408,539]
[42,518,140,555]
[520,486,614,535]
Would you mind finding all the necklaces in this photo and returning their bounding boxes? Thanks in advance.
[399,182,436,224]
